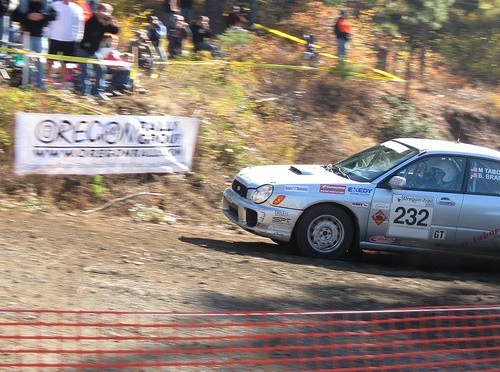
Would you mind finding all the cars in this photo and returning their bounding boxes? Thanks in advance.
[222,137,500,268]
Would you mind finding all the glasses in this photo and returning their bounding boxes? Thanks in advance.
[432,171,442,177]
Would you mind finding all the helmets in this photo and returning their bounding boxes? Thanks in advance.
[431,161,461,183]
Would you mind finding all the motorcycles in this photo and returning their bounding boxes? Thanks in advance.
[130,30,153,72]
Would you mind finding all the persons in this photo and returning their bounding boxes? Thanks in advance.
[334,8,353,57]
[0,0,257,100]
[431,159,460,190]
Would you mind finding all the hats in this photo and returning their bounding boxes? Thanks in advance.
[92,4,113,17]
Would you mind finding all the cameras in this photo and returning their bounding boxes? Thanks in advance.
[104,12,111,20]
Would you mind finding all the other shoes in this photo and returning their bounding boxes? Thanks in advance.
[0,0,226,103]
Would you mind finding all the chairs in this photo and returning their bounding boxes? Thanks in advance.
[415,166,445,189]
